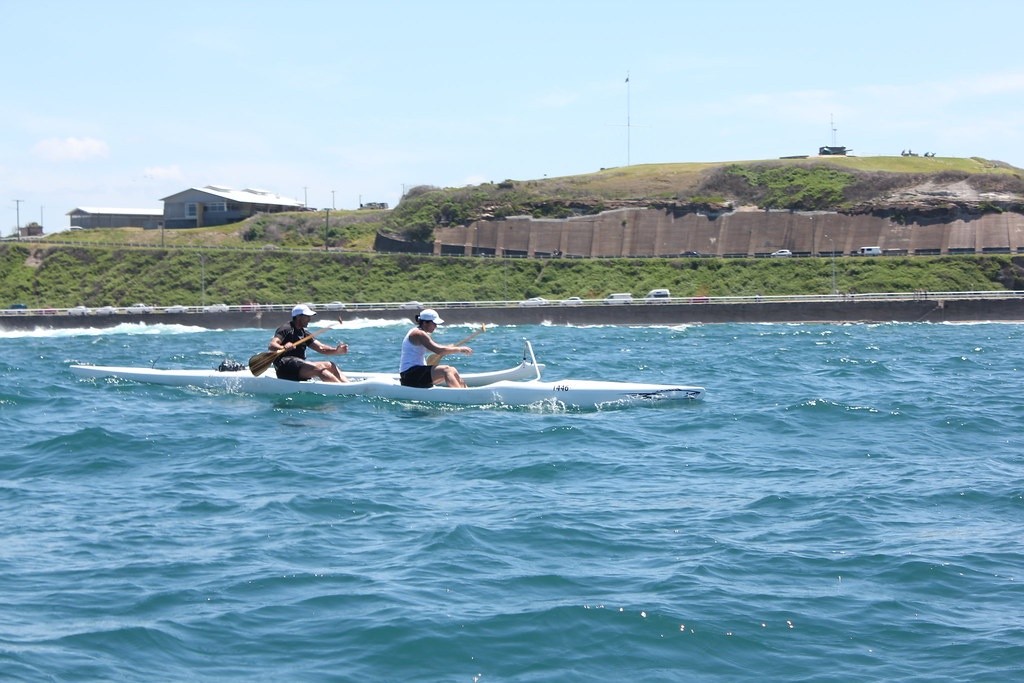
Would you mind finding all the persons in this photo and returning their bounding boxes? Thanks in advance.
[398,308,473,390]
[269,304,353,384]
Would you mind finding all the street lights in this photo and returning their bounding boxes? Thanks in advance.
[825,233,837,293]
[196,251,205,312]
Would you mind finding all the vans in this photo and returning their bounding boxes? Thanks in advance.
[603,291,633,306]
[857,246,883,256]
[643,288,672,303]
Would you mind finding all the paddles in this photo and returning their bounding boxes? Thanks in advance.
[425,323,486,368]
[249,316,343,377]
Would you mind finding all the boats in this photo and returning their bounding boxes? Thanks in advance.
[70,339,708,408]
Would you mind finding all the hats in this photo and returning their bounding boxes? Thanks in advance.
[291,304,317,317]
[417,308,444,325]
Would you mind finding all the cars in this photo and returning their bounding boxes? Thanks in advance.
[520,296,549,308]
[535,296,549,306]
[8,295,348,317]
[769,249,792,259]
[399,299,425,310]
[687,295,712,304]
[560,296,582,306]
